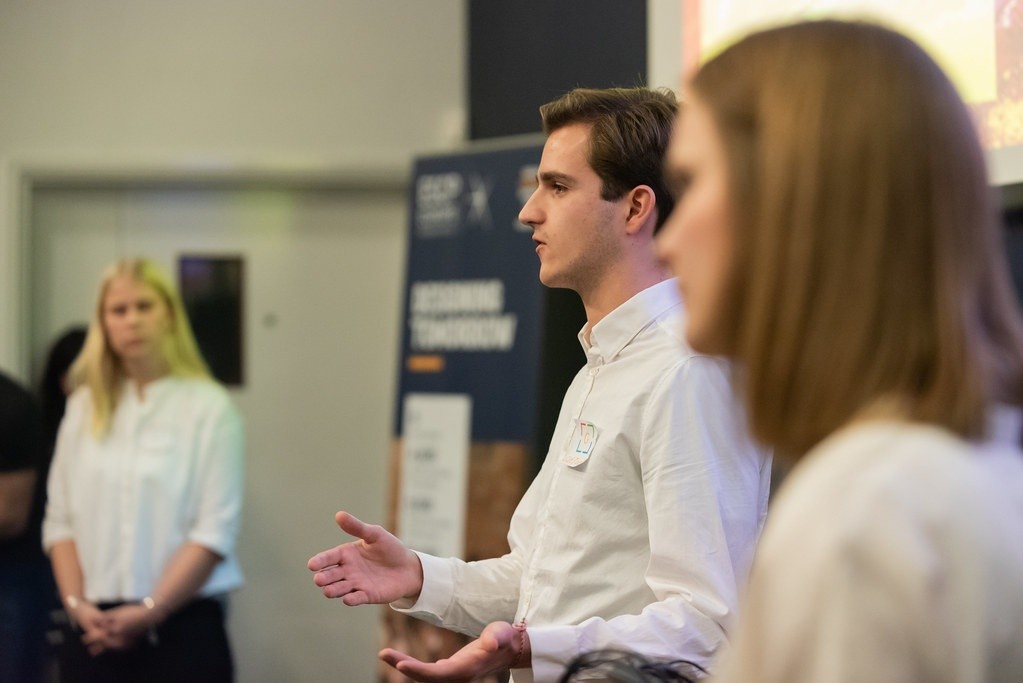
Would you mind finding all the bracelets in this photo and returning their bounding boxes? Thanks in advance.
[504,611,527,675]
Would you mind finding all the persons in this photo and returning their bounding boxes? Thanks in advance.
[40,255,241,683]
[645,17,1023,679]
[0,321,89,683]
[306,85,780,683]
